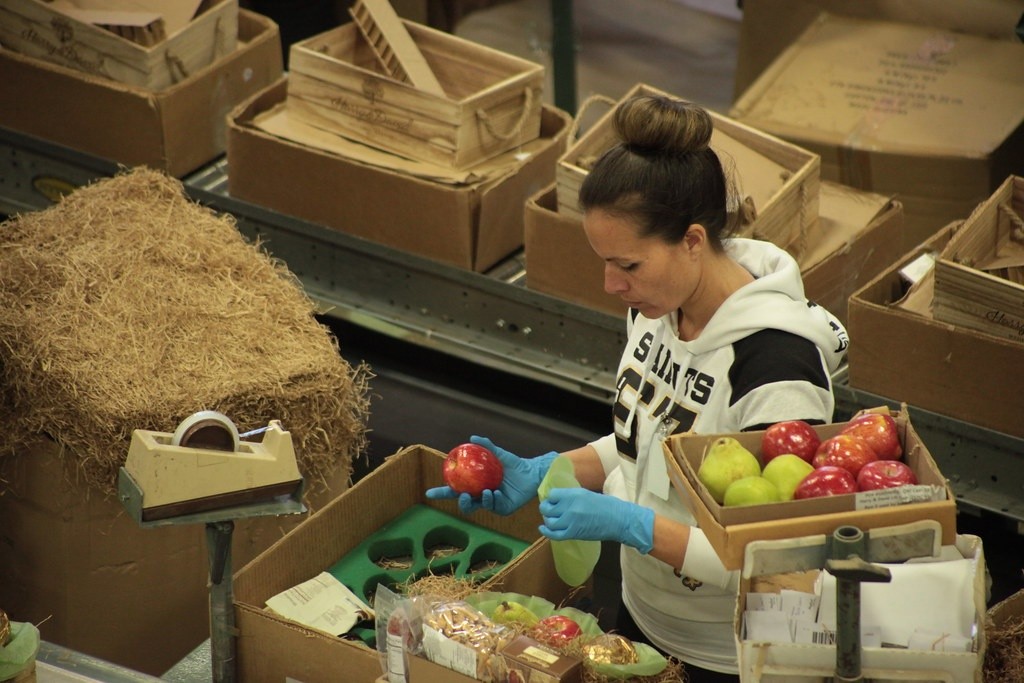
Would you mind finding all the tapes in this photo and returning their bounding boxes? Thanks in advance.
[172,410,239,452]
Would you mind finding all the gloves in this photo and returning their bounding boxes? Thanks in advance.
[426,435,559,516]
[539,487,655,555]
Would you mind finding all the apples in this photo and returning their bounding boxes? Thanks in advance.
[813,436,879,480]
[764,421,820,466]
[837,413,901,460]
[534,616,581,647]
[794,465,857,499]
[857,460,917,491]
[442,443,502,495]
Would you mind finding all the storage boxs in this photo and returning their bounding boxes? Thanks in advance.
[732,0,1024,102]
[730,9,1024,251]
[522,181,904,335]
[670,416,948,528]
[735,532,989,683]
[225,77,573,273]
[555,81,821,251]
[209,444,545,683]
[931,174,1024,341]
[406,541,621,683]
[285,17,547,173]
[663,402,957,571]
[498,635,584,683]
[845,218,1024,437]
[0,0,239,91]
[0,436,351,677]
[0,7,285,180]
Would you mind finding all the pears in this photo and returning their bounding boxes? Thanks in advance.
[698,437,761,503]
[491,600,539,635]
[725,476,780,506]
[762,455,816,501]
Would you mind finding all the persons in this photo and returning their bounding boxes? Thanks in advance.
[428,96,832,682]
